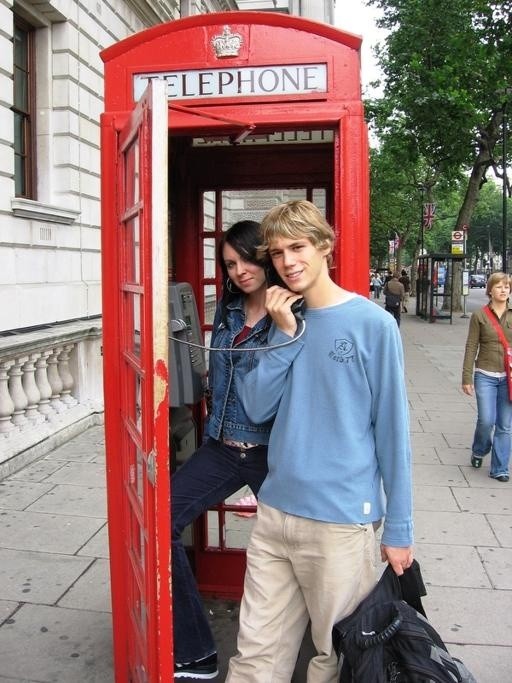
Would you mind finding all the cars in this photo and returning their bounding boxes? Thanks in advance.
[437,265,447,285]
[469,275,486,288]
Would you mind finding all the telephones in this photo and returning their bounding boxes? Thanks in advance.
[263,265,306,310]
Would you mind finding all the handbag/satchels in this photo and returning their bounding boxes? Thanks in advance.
[384,295,401,311]
[503,345,512,403]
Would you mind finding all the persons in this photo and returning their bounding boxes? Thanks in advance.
[460,270,511,482]
[369,272,383,300]
[398,269,411,312]
[383,270,404,327]
[382,270,393,288]
[224,196,415,682]
[162,216,277,681]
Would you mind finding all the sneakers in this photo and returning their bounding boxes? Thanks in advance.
[171,651,219,681]
[496,476,510,482]
[471,454,483,468]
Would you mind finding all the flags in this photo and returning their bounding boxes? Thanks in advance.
[422,203,436,229]
[392,232,401,249]
[387,240,395,253]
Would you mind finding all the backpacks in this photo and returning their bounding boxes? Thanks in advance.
[331,559,480,683]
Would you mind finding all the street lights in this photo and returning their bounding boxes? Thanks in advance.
[418,182,423,255]
